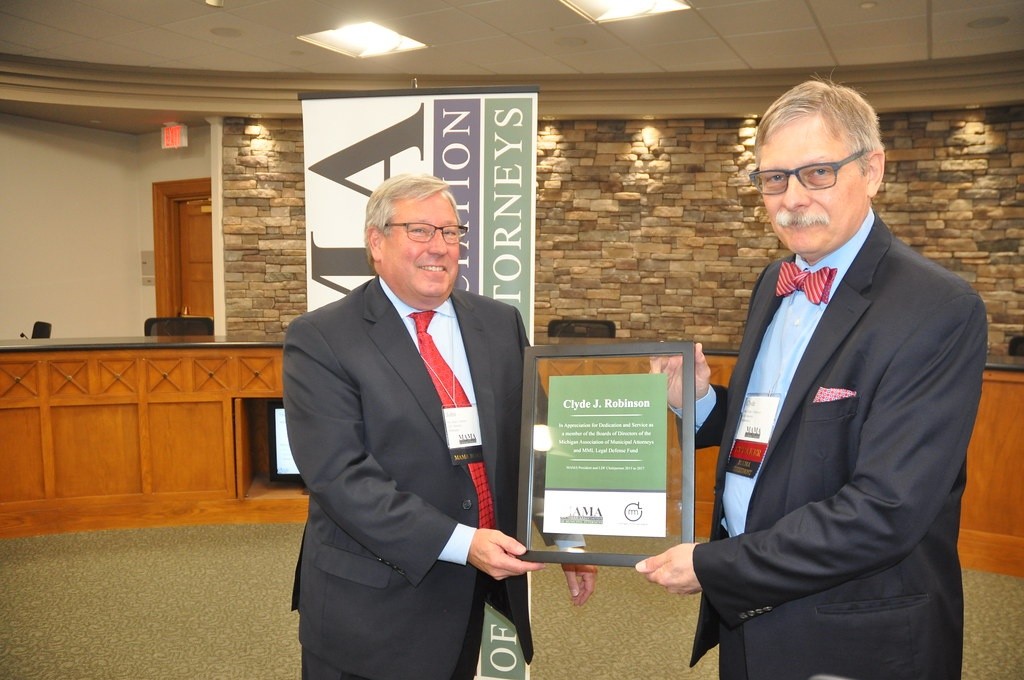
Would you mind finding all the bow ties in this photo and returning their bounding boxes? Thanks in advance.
[773,260,836,306]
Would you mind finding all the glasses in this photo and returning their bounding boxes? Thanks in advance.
[384,222,468,244]
[748,150,863,196]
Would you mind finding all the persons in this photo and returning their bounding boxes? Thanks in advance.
[282,174,599,680]
[636,79,989,679]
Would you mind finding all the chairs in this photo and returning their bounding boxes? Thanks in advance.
[548,320,616,338]
[31,321,52,338]
[145,317,213,336]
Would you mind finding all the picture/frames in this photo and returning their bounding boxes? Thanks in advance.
[514,339,697,568]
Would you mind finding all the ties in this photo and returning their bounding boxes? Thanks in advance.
[407,310,497,528]
